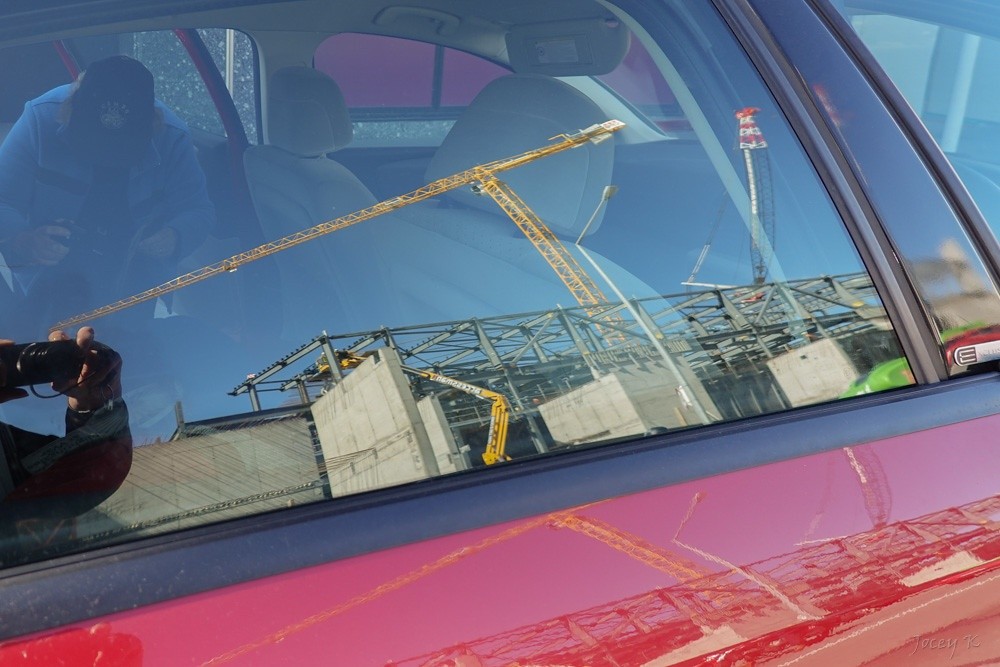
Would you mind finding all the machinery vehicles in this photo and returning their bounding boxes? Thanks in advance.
[315,351,512,465]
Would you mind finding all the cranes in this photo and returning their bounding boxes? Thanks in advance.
[46,119,637,348]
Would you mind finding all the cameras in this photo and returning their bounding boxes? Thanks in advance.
[0,339,84,389]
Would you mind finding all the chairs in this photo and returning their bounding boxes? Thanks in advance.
[367,73,680,343]
[238,70,385,298]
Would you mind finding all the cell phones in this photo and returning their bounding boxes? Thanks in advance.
[53,219,109,255]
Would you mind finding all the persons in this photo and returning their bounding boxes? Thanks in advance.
[0,327,133,522]
[0,55,215,270]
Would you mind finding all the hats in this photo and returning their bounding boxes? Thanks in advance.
[67,56,154,164]
